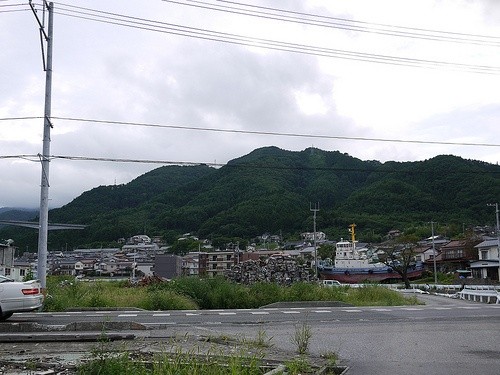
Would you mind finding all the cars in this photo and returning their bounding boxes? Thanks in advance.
[322,280,352,289]
[0,273,44,322]
